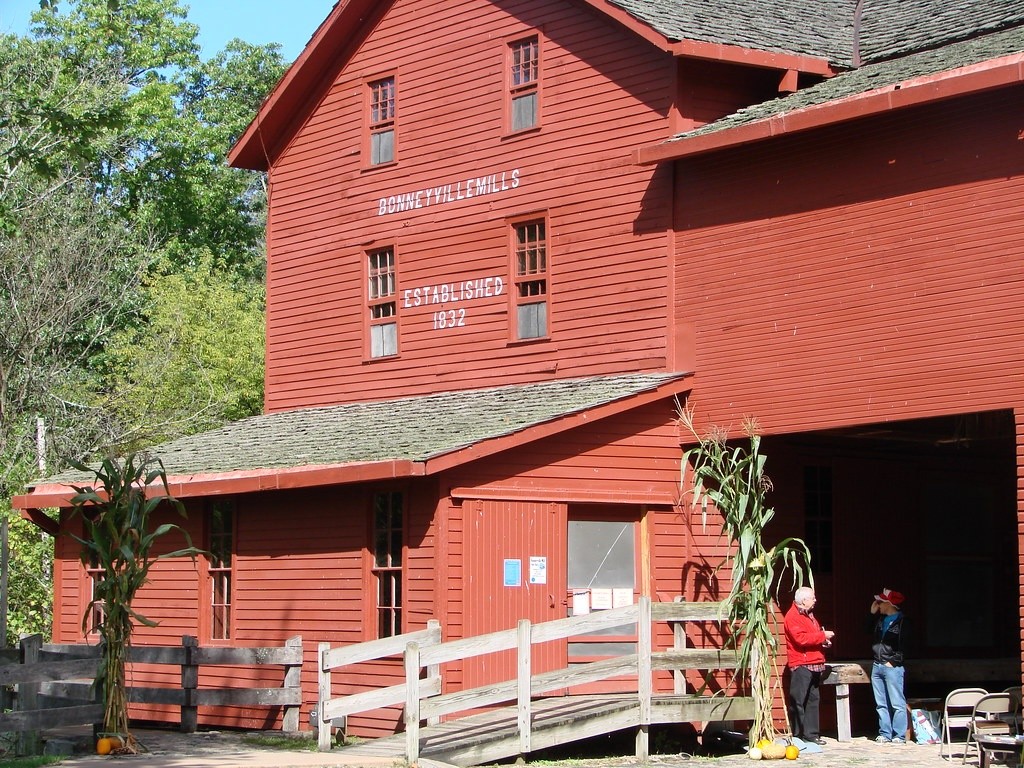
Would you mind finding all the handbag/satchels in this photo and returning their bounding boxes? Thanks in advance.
[906,704,943,745]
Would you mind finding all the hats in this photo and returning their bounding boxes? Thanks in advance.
[874,589,905,609]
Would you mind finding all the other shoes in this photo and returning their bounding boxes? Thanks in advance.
[891,737,906,743]
[875,736,892,743]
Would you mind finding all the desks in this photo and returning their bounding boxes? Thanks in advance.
[973,734,1023,767]
[818,660,882,743]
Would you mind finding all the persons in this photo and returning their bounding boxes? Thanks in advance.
[861,587,912,743]
[783,587,835,745]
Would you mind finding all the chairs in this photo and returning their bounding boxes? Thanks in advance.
[938,684,1023,767]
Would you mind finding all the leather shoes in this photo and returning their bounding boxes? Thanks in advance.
[795,734,807,742]
[802,736,826,745]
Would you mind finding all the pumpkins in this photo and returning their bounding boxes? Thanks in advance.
[97,737,122,754]
[749,739,800,759]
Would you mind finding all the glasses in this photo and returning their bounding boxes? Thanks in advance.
[877,600,889,606]
